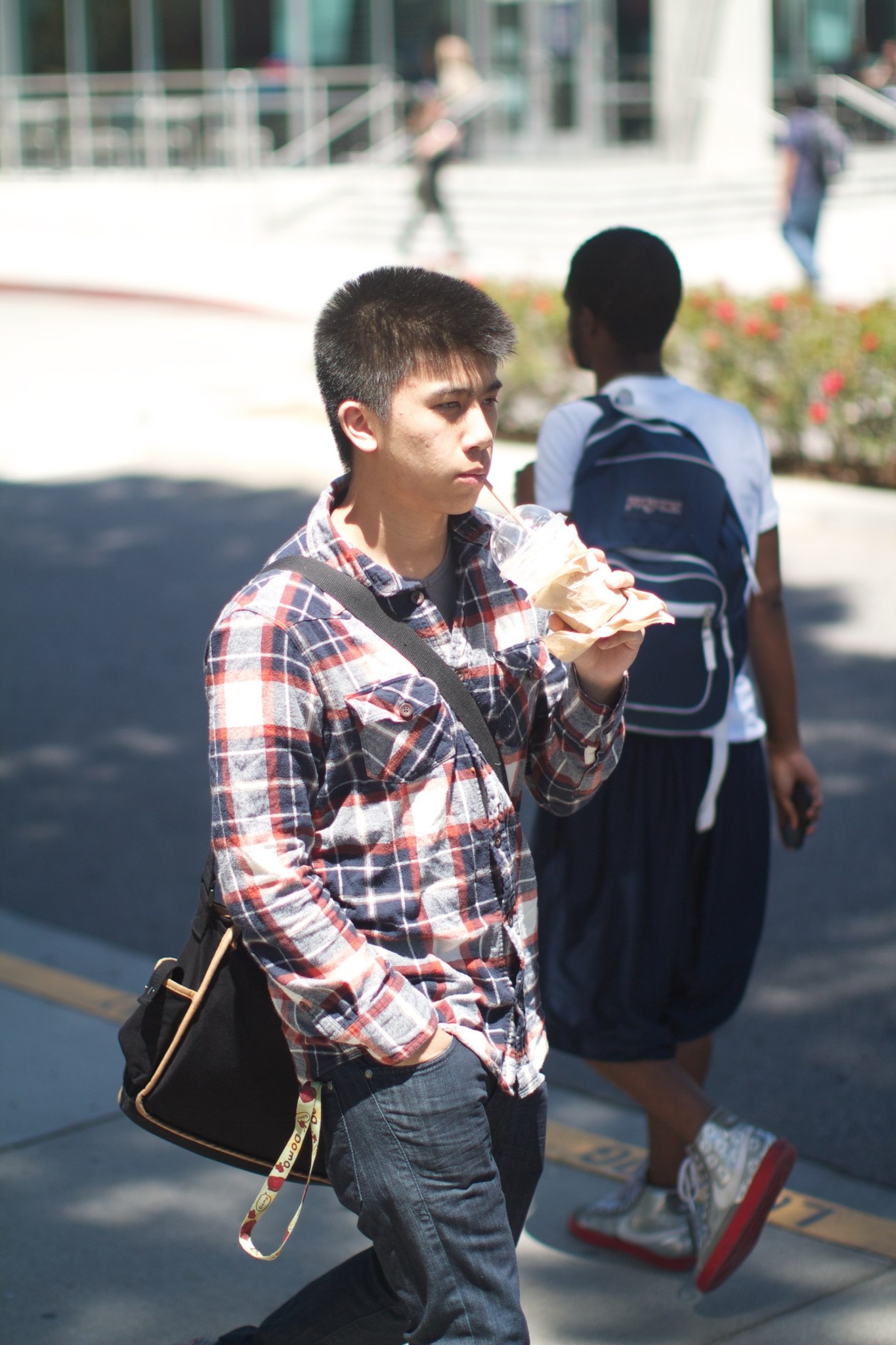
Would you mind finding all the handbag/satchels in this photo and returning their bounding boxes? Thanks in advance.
[116,866,330,1260]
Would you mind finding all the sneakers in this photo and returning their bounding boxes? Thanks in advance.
[676,1105,796,1295]
[570,1181,700,1272]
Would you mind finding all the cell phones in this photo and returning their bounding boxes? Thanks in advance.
[777,776,815,852]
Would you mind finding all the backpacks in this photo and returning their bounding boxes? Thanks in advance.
[570,396,751,729]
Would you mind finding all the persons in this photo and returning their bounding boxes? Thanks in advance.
[780,85,844,299]
[386,29,484,262]
[515,228,825,1295]
[204,272,644,1345]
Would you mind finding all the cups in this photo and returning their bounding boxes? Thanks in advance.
[488,504,627,635]
